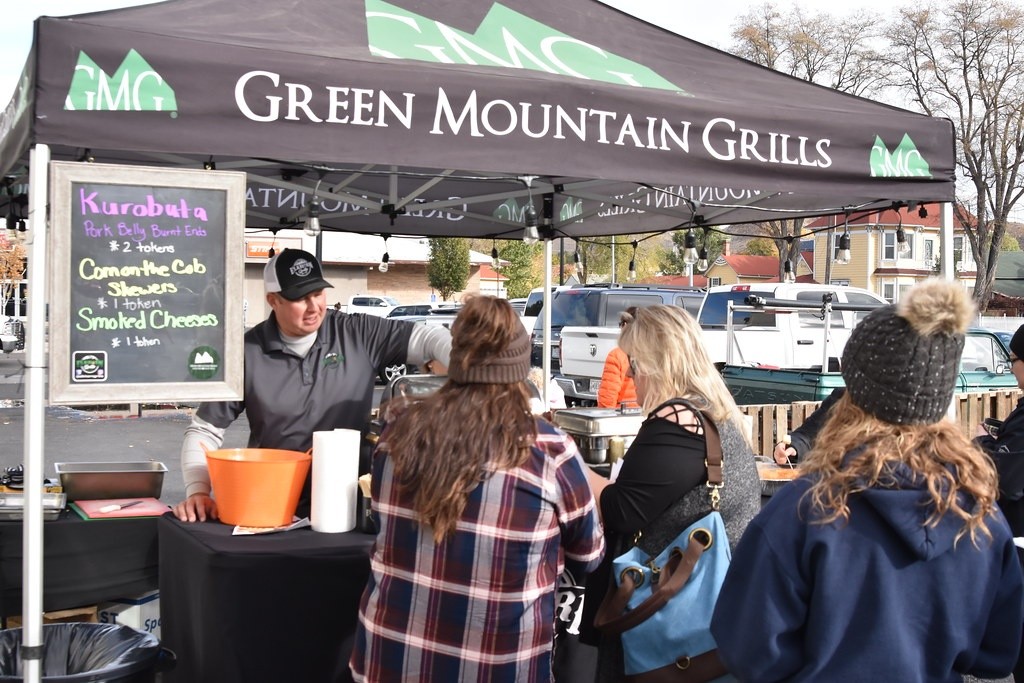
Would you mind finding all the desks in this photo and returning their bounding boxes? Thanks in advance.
[0,497,174,624]
[156,513,382,683]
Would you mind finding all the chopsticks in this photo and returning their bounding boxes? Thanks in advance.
[359,474,372,498]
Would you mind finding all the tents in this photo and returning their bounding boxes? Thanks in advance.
[0,0,956,683]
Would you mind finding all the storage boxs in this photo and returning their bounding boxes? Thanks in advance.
[99,589,162,648]
[6,606,97,629]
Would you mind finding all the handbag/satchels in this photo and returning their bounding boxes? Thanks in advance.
[594,402,732,683]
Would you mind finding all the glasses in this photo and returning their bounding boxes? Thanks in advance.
[1006,358,1020,368]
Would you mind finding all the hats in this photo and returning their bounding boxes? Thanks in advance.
[1009,324,1024,362]
[449,311,531,384]
[841,277,975,426]
[264,248,335,302]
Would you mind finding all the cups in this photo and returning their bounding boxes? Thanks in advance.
[358,497,379,534]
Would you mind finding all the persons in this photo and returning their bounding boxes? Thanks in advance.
[773,386,846,467]
[968,324,1024,569]
[597,307,640,409]
[173,248,453,522]
[578,305,762,683]
[348,294,605,683]
[707,276,1024,683]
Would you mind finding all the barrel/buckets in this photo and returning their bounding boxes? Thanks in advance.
[205,448,312,528]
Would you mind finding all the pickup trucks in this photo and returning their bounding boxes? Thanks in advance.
[326,293,403,318]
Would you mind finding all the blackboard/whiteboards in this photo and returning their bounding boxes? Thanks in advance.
[49,158,247,404]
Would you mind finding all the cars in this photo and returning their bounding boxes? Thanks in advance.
[382,274,1024,426]
